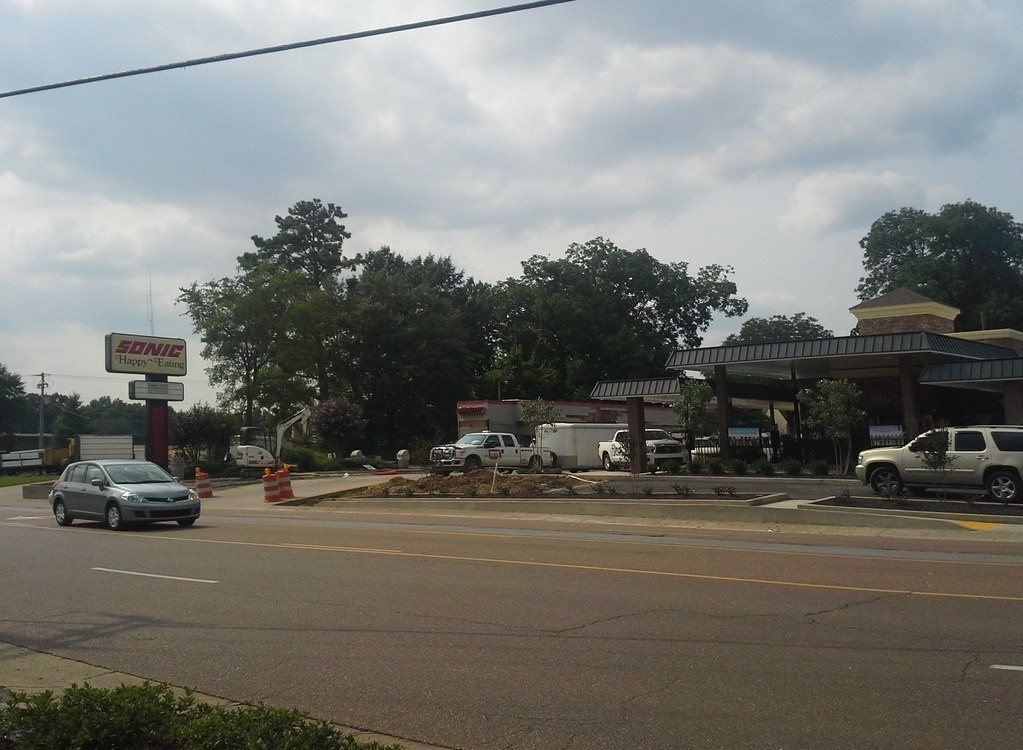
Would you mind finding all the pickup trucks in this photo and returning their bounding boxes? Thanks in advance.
[597,428,690,471]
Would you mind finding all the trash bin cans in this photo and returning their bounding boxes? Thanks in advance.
[167,457,186,480]
[350,450,363,458]
[397,450,410,469]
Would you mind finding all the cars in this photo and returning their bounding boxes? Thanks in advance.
[47,458,203,531]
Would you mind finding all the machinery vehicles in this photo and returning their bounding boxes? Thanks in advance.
[223,403,314,480]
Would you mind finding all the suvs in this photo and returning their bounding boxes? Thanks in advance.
[855,424,1022,503]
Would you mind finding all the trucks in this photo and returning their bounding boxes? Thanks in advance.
[535,421,629,471]
[427,429,556,476]
